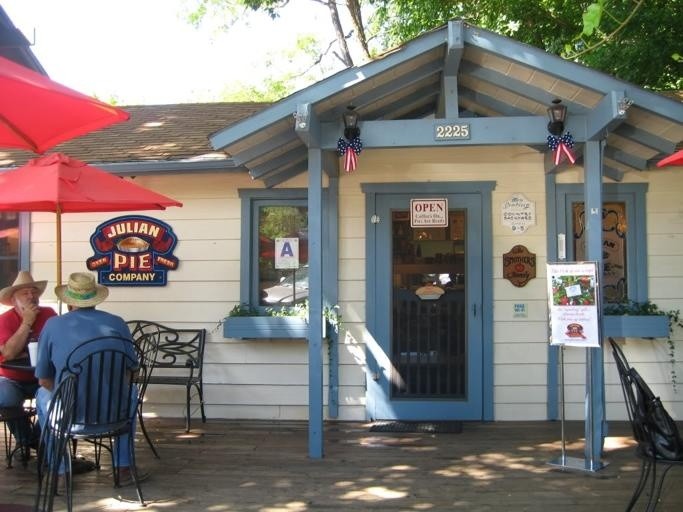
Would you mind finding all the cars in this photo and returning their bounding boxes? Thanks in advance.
[261,265,309,305]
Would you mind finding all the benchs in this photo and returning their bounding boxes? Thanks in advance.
[131,322,206,433]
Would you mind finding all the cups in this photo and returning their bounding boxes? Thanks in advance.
[28,342,38,367]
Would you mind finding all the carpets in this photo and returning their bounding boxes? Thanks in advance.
[368,418,461,434]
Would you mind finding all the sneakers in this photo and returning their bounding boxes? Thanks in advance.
[13,444,30,461]
[118,466,151,486]
[43,474,65,496]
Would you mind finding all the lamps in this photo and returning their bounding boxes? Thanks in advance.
[547,97,567,135]
[341,103,360,140]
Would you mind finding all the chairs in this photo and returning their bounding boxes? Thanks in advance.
[605,335,682,510]
[0,320,159,512]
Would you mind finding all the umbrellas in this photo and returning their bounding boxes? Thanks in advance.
[656,149,683,167]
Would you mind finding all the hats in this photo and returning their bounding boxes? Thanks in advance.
[0,271,48,306]
[55,272,109,308]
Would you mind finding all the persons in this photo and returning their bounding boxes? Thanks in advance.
[0,271,151,486]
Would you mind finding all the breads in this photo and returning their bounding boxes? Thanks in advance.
[118,236,149,249]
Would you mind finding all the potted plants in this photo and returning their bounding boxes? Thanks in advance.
[222,301,345,340]
[600,299,682,395]
